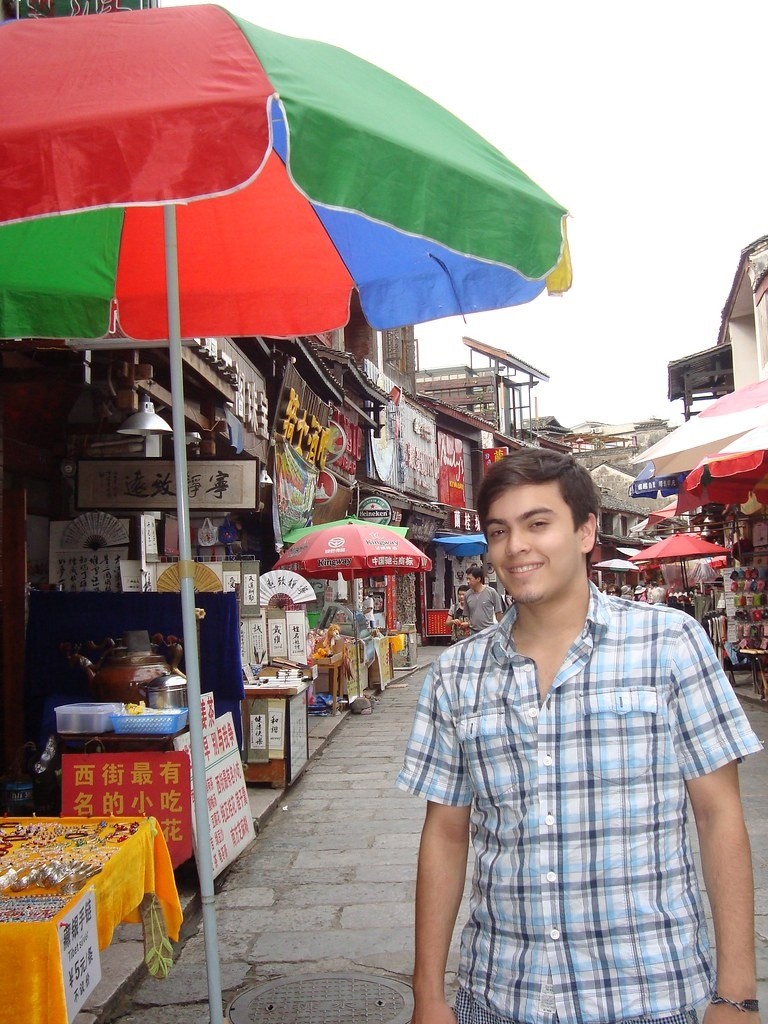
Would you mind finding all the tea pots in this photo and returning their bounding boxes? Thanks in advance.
[68,640,184,707]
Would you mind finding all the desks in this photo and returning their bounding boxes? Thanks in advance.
[317,660,344,717]
[0,816,183,1024]
[240,682,309,789]
[61,723,190,752]
[740,648,768,700]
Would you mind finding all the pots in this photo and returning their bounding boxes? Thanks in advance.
[138,672,190,725]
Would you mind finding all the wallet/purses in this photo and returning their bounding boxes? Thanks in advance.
[730,566,768,650]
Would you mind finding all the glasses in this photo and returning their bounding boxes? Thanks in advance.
[607,591,616,595]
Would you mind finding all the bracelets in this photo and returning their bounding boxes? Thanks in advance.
[710,992,759,1013]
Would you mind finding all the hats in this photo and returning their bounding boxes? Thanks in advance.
[621,586,630,594]
[634,586,646,594]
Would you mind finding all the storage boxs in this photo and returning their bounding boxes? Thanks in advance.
[315,652,343,665]
[54,703,123,734]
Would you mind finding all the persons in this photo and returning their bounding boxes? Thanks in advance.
[363,597,375,629]
[393,448,764,1024]
[446,567,515,643]
[598,580,668,605]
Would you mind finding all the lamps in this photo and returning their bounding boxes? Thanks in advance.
[260,463,274,485]
[170,429,201,444]
[116,393,173,436]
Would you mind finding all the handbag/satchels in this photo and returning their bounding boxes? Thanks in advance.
[717,593,726,609]
[190,515,279,575]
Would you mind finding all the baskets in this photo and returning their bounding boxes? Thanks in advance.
[109,708,187,735]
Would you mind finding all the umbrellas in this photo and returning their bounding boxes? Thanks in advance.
[270,521,433,698]
[589,377,768,590]
[0,6,573,1024]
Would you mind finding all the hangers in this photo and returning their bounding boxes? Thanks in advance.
[703,610,723,618]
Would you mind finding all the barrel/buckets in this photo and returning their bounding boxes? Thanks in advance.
[306,612,320,629]
